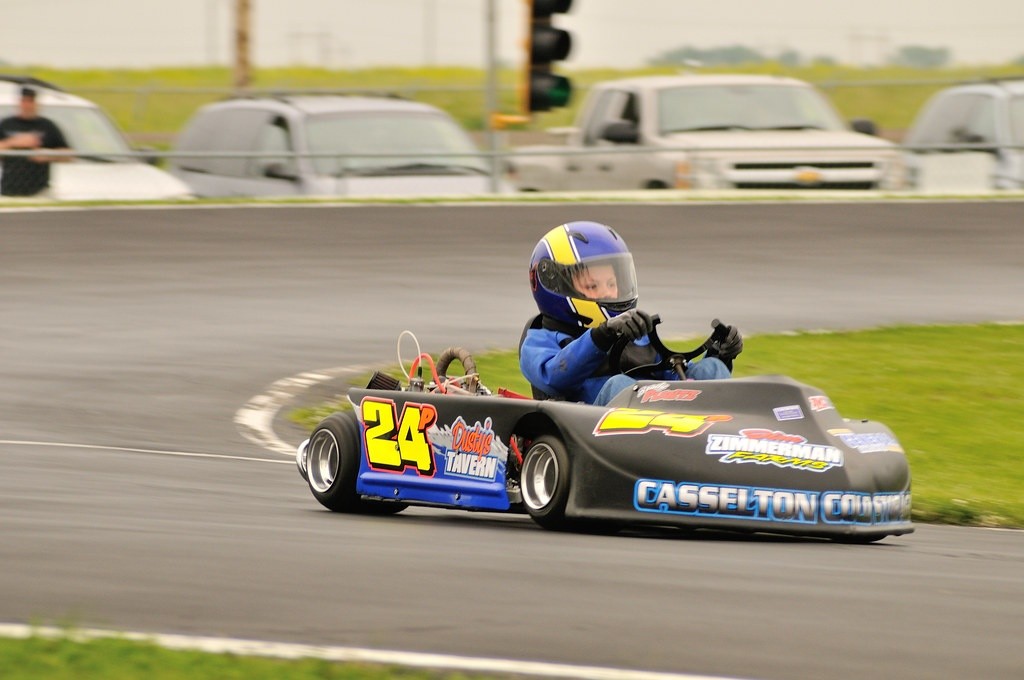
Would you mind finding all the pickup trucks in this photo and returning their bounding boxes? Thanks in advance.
[500,74,920,195]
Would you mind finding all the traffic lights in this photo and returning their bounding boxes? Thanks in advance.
[521,0,572,115]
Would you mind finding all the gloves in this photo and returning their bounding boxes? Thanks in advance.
[599,308,653,343]
[716,326,743,359]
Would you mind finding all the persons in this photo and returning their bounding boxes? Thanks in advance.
[517,222,744,407]
[0,87,73,200]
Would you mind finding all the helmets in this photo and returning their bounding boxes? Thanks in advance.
[528,221,639,337]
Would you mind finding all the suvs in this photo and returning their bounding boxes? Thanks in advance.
[170,91,517,197]
[0,75,199,201]
[903,78,1024,190]
